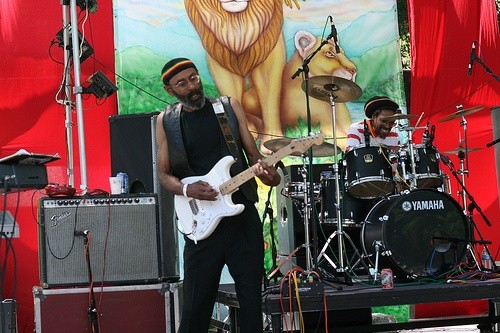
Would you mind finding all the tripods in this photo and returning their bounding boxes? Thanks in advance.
[265,34,378,292]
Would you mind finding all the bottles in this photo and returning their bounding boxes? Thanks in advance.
[482,246,492,273]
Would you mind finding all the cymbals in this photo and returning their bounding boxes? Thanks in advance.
[441,104,486,122]
[262,137,342,158]
[442,147,484,154]
[300,75,363,103]
[380,114,417,121]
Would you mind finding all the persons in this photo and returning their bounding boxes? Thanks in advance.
[156,57,281,333]
[347,95,401,193]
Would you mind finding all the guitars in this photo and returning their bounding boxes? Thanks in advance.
[173,131,326,242]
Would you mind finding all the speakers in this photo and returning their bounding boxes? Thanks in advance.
[108,111,182,281]
[38,193,163,286]
[276,163,349,256]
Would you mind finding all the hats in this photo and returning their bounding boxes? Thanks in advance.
[364,96,399,119]
[161,58,198,86]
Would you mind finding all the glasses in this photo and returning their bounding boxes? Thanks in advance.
[378,115,397,127]
[164,75,200,90]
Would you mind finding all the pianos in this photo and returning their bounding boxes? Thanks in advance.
[0,163,50,194]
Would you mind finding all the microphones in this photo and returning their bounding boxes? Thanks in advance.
[468,41,476,76]
[330,16,340,53]
[74,230,90,236]
[423,123,430,143]
[278,160,290,181]
[363,119,371,146]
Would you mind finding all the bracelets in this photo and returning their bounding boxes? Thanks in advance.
[182,183,189,198]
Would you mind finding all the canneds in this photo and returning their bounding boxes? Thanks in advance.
[381,269,394,289]
[116,172,128,194]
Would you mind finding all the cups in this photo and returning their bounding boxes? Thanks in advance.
[109,177,122,195]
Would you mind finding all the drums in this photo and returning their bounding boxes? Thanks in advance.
[360,187,472,280]
[399,143,443,190]
[344,143,397,200]
[317,169,371,227]
